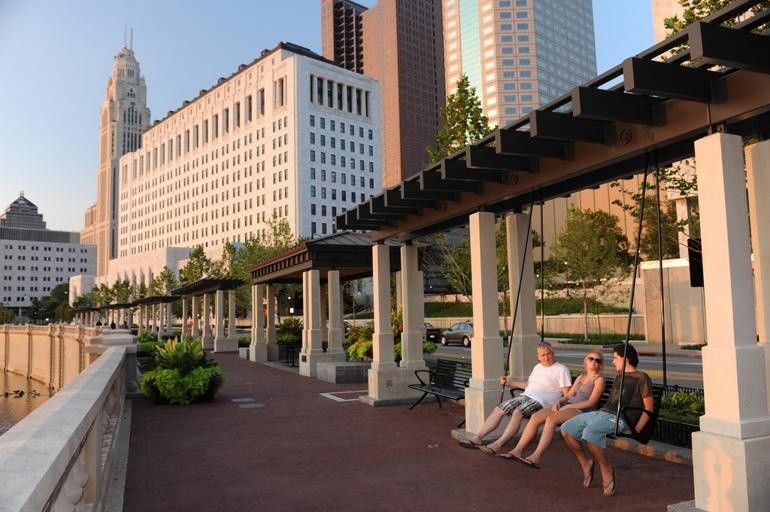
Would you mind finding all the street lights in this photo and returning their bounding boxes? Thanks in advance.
[563,259,571,298]
[352,291,362,326]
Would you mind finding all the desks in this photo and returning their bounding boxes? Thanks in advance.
[282,345,302,367]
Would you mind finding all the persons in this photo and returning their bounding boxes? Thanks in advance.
[560,343,655,497]
[456,340,572,456]
[500,349,607,470]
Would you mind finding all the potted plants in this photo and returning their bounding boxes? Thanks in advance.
[140,334,224,405]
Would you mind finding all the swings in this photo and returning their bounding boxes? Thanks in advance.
[499,150,666,444]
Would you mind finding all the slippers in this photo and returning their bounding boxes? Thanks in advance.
[459,438,483,449]
[519,457,541,470]
[498,451,523,461]
[583,458,594,489]
[479,445,501,456]
[602,464,619,496]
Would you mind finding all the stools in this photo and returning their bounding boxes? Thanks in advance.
[280,349,293,363]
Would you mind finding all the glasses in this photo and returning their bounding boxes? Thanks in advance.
[587,357,601,364]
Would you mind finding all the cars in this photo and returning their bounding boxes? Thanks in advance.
[425,322,473,347]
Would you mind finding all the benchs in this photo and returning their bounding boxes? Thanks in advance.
[510,376,664,445]
[408,359,472,427]
